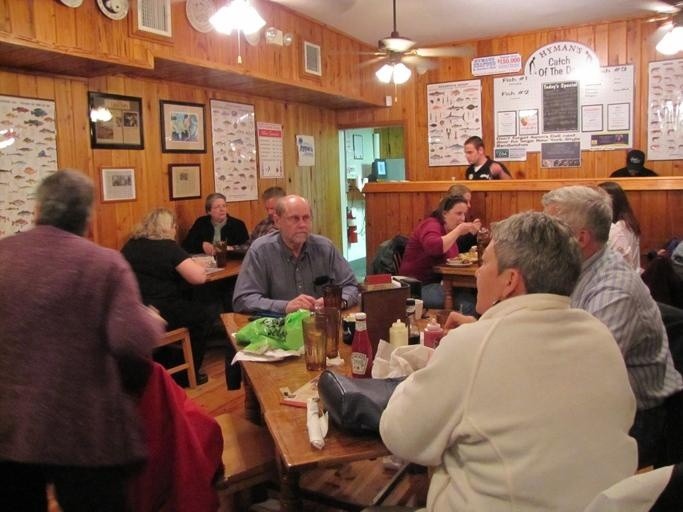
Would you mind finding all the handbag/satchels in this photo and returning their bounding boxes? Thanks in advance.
[318,369,404,438]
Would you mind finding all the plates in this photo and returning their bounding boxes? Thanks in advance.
[446,261,472,268]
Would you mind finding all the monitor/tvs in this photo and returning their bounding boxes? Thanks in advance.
[368,159,388,181]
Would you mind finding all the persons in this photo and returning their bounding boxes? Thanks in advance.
[249,184,288,249]
[2,167,169,511]
[398,193,481,311]
[608,148,659,177]
[597,180,641,272]
[542,183,682,469]
[231,195,359,316]
[376,207,640,511]
[121,207,209,389]
[181,193,249,262]
[449,185,473,209]
[463,135,514,181]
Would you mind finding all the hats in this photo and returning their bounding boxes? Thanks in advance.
[626,150,645,169]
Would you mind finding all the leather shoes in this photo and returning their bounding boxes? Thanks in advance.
[198,373,208,384]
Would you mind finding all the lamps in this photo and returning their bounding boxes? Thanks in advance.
[375,62,411,85]
[655,26,683,55]
[208,0,266,36]
[265,26,294,47]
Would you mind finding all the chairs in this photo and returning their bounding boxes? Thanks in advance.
[641,237,677,300]
[131,353,277,512]
[143,326,197,389]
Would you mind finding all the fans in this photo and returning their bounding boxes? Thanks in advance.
[327,0,473,75]
[641,0,683,45]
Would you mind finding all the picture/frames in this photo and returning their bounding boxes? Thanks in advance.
[160,99,207,153]
[88,90,145,150]
[168,163,202,201]
[99,165,138,204]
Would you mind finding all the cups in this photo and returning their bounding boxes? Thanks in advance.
[475,230,487,267]
[301,315,325,372]
[341,314,355,345]
[321,286,342,324]
[314,306,339,359]
[215,241,226,267]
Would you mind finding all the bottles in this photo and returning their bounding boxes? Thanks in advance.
[423,319,443,348]
[404,297,420,345]
[349,313,372,378]
[388,319,408,349]
